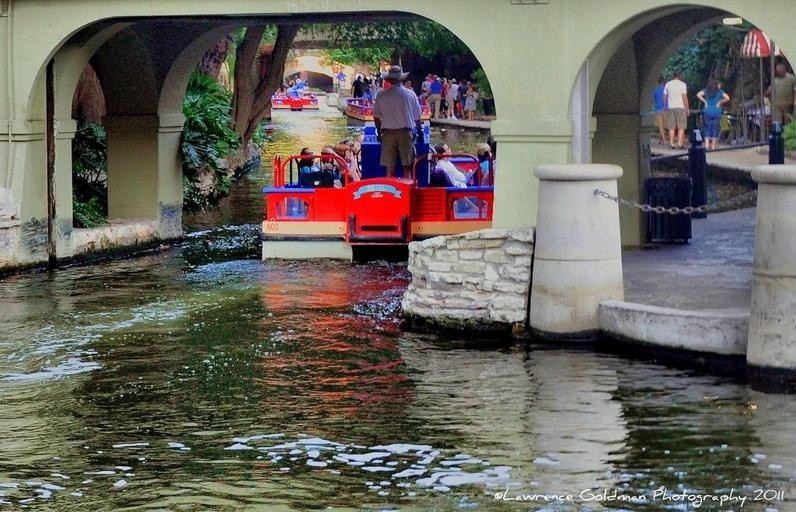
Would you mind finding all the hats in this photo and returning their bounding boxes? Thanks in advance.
[384,66,408,81]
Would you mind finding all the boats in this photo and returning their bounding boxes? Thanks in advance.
[271,91,319,111]
[345,98,431,120]
[258,152,496,261]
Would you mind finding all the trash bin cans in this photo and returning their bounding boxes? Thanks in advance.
[647,176,693,244]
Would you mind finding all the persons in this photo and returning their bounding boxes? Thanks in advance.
[298,140,361,188]
[764,64,796,125]
[655,77,666,146]
[695,80,730,150]
[663,71,691,150]
[336,70,346,80]
[272,79,310,98]
[483,95,494,115]
[373,65,422,180]
[430,139,497,187]
[404,70,479,121]
[351,71,391,107]
